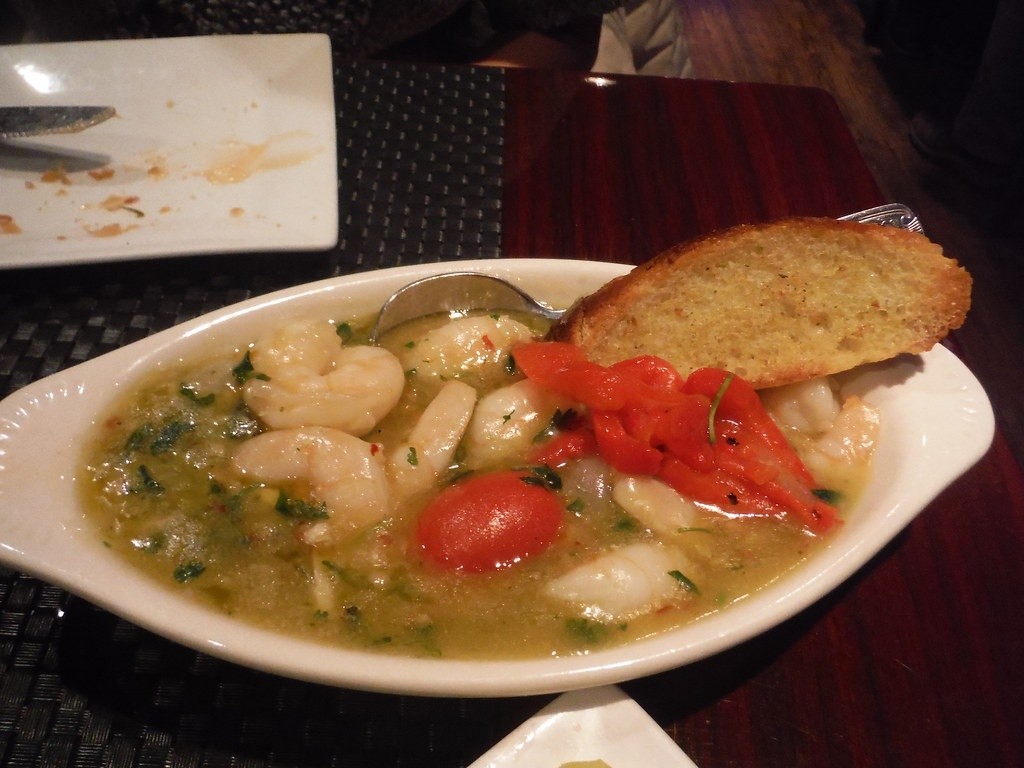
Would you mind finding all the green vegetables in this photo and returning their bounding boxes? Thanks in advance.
[117,320,844,660]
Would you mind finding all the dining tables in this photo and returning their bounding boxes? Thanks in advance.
[0,56,1024,768]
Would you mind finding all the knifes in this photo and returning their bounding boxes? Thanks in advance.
[0,103,117,140]
[0,140,113,175]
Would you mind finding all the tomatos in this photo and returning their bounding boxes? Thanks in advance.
[416,469,564,576]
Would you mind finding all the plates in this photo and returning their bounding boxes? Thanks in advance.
[0,32,339,271]
[460,684,701,768]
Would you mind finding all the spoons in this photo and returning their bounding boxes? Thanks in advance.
[368,201,927,340]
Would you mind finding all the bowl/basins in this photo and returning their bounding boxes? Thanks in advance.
[0,257,998,699]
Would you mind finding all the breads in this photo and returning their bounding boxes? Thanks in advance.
[540,215,972,392]
